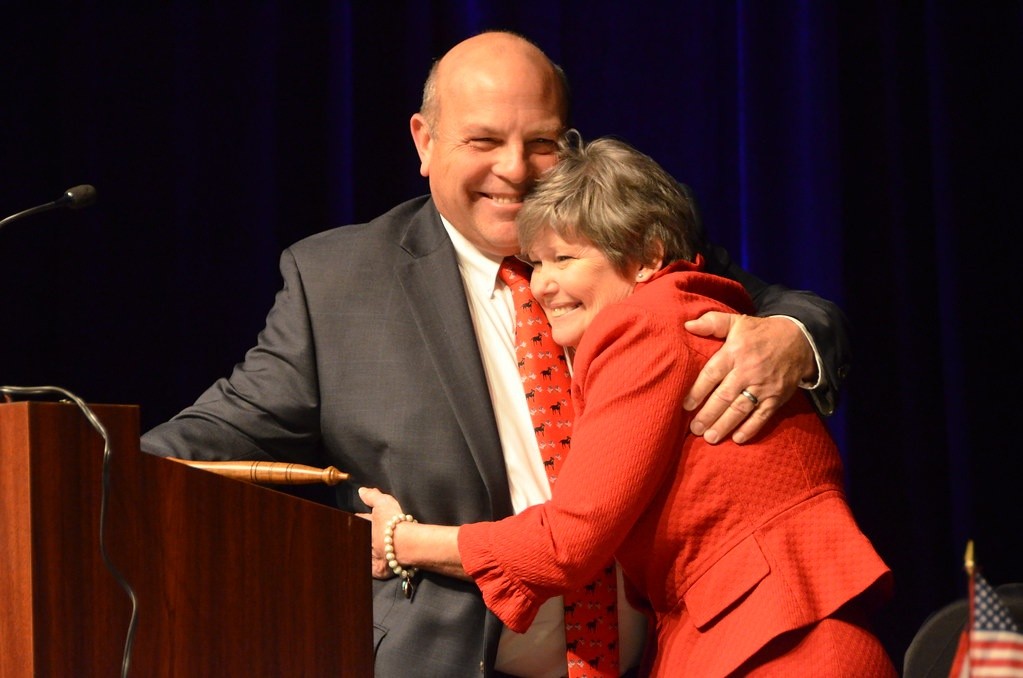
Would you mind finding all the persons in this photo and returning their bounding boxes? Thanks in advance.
[356,130,896,678]
[139,30,842,678]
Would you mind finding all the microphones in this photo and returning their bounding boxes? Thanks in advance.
[0,185,96,228]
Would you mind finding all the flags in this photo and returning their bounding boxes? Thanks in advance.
[946,571,1023,678]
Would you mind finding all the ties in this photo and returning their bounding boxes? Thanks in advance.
[498,259,622,678]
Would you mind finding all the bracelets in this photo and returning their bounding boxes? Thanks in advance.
[383,514,418,603]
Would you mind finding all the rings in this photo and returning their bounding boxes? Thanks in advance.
[743,391,758,407]
